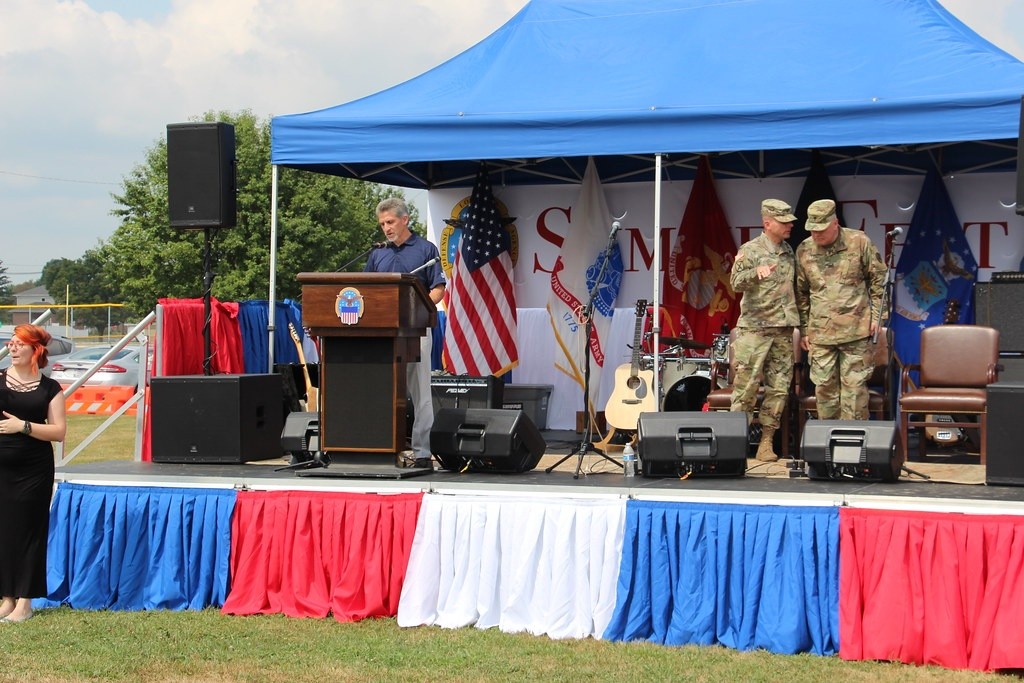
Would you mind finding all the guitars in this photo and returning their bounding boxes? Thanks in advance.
[288,321,322,414]
[604,299,656,430]
[925,299,967,444]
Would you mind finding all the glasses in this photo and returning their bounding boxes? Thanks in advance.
[6,341,30,348]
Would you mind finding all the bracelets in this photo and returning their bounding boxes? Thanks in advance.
[22,421,32,435]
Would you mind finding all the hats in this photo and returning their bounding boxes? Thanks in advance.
[804,199,836,231]
[760,198,798,223]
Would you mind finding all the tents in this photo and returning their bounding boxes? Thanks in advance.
[265,0,1024,407]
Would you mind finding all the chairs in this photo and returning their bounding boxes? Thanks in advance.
[707,327,802,458]
[799,327,894,460]
[899,324,1000,463]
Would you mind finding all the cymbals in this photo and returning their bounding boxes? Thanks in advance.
[659,336,711,350]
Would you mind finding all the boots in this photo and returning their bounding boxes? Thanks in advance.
[755,426,779,461]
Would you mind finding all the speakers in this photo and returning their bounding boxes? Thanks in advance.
[280,411,324,463]
[799,420,905,481]
[972,272,1024,486]
[151,374,285,463]
[166,122,236,229]
[636,411,749,478]
[430,376,553,474]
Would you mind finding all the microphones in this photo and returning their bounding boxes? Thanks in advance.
[371,241,392,248]
[887,227,904,236]
[609,221,621,238]
[724,320,730,334]
[410,257,441,274]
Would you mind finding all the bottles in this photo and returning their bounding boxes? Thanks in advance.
[623,443,634,477]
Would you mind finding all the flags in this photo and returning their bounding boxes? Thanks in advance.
[784,149,848,253]
[664,153,743,360]
[441,164,518,376]
[891,157,980,390]
[545,157,625,400]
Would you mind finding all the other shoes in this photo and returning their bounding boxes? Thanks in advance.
[415,458,434,475]
[0,601,34,623]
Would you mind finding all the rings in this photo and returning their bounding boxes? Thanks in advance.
[760,271,762,272]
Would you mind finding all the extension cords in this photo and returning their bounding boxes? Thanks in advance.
[789,468,807,477]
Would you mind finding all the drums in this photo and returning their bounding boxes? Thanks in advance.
[662,375,729,413]
[659,359,711,410]
[712,333,731,365]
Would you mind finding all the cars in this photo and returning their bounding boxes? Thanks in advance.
[0,326,77,378]
[51,346,151,395]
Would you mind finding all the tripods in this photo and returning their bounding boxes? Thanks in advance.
[274,335,326,472]
[546,234,623,479]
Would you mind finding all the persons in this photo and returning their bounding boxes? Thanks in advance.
[730,198,889,460]
[0,323,67,623]
[364,199,447,471]
